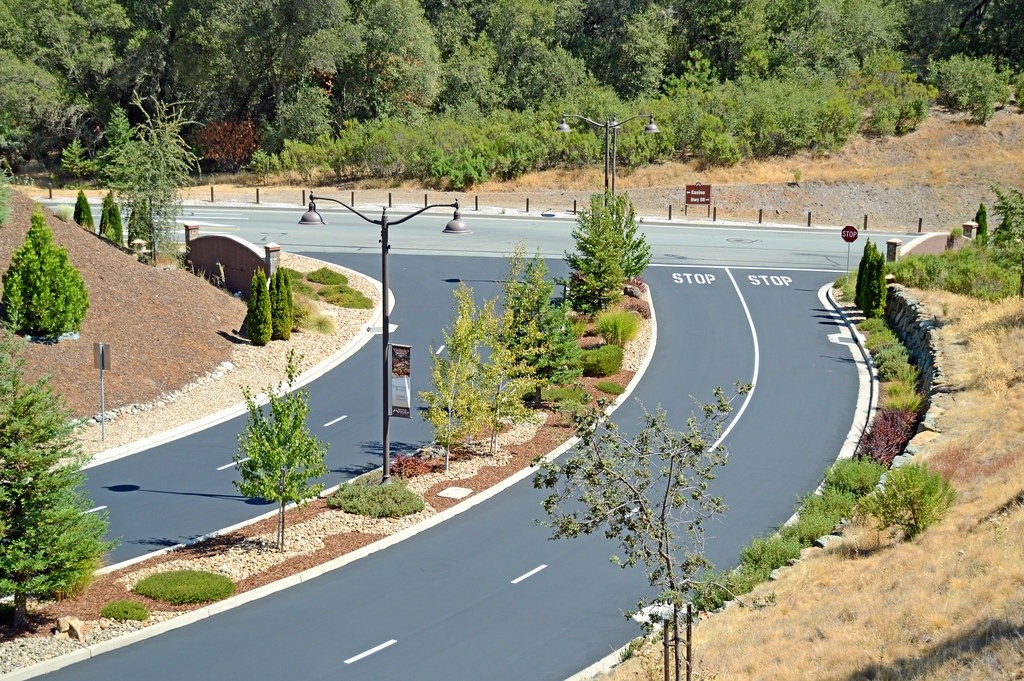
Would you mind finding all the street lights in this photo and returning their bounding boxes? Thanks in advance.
[297,191,471,487]
[555,114,661,223]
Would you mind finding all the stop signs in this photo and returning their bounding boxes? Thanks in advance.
[842,225,858,243]
